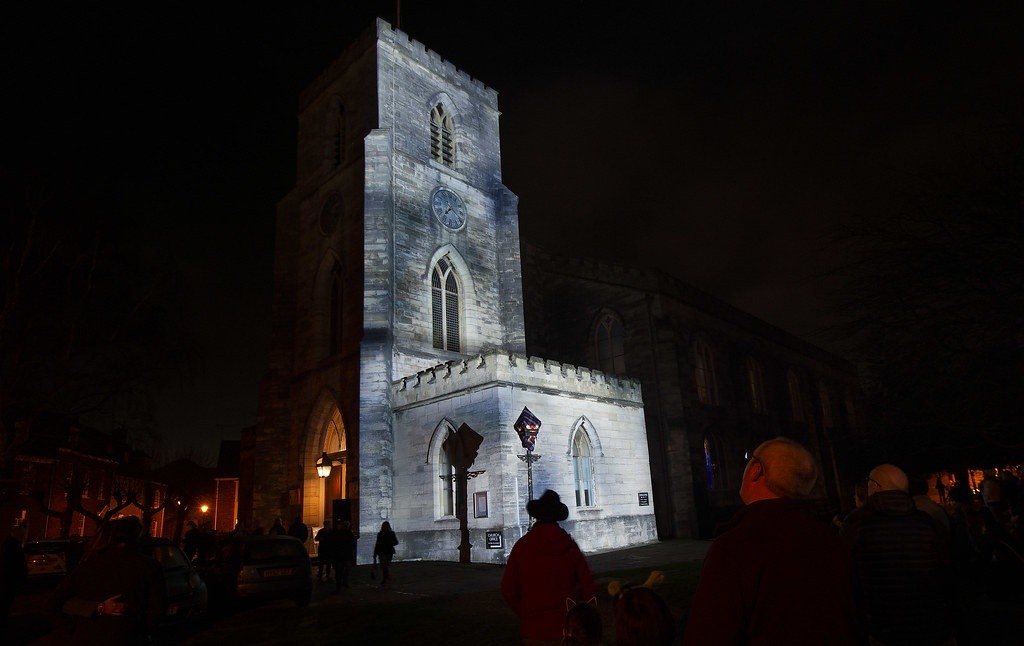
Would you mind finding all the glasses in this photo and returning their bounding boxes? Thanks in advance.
[744,448,763,476]
[866,475,882,488]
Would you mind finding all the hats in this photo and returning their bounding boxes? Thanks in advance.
[526,490,569,521]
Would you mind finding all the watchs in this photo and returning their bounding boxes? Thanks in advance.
[98,602,104,616]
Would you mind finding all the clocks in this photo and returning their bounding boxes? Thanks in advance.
[430,188,467,232]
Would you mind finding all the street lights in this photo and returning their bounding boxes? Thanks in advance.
[201,504,209,524]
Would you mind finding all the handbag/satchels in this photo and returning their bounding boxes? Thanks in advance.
[371,555,378,579]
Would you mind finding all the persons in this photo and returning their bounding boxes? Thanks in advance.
[501,490,602,646]
[47,515,164,646]
[373,522,398,585]
[683,437,1024,646]
[315,521,354,578]
[268,517,308,544]
[0,524,28,634]
[612,578,665,646]
